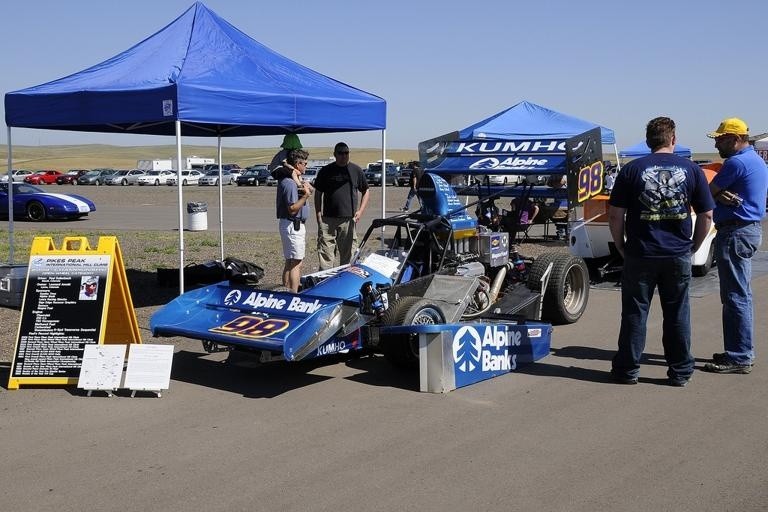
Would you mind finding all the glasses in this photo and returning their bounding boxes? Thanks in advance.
[334,148,352,156]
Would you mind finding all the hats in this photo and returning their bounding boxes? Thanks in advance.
[278,131,305,150]
[706,118,750,141]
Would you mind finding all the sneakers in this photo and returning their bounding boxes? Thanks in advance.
[702,350,755,375]
[669,375,692,387]
[599,363,643,386]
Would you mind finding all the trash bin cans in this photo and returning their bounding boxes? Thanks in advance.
[187,201,208,231]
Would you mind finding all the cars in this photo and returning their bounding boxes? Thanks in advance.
[474,161,625,188]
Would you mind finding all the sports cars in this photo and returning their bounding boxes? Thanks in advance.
[1,182,98,223]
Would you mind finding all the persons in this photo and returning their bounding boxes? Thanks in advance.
[398,160,423,213]
[473,194,568,242]
[269,133,314,197]
[603,115,718,388]
[607,168,619,197]
[275,149,314,294]
[314,142,370,272]
[702,116,767,375]
